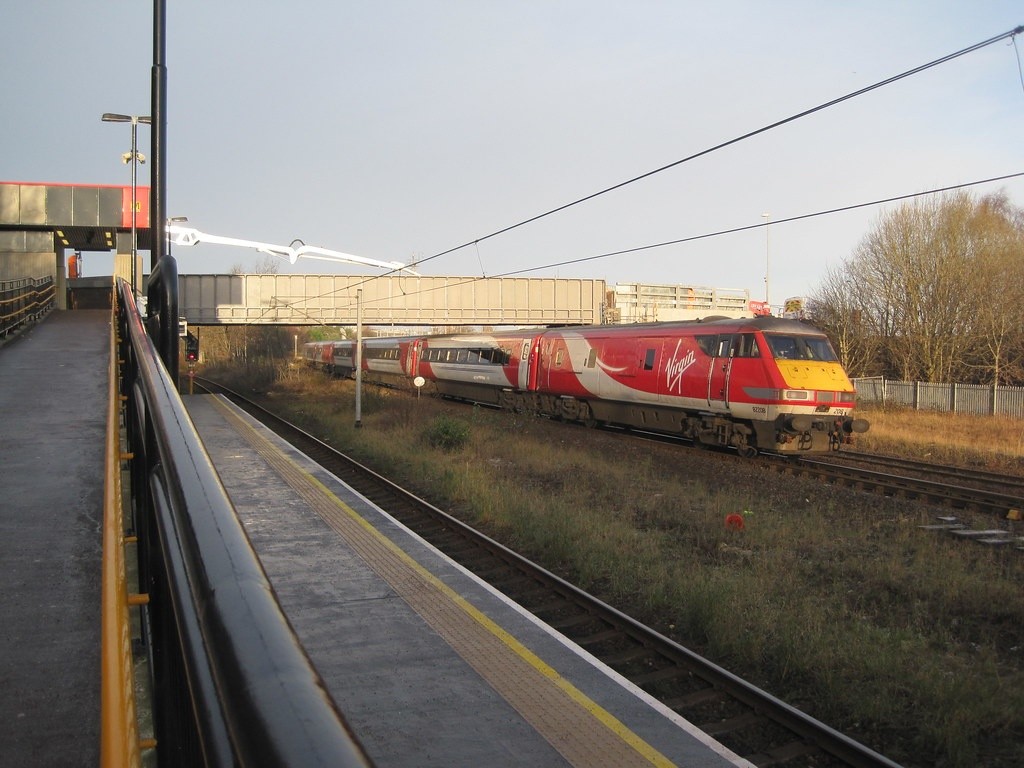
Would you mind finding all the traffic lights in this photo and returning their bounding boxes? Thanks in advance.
[186,338,199,362]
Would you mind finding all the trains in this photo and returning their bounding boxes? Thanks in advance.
[303,312,870,459]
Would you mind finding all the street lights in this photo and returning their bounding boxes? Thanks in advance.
[101,112,152,304]
[761,213,770,305]
